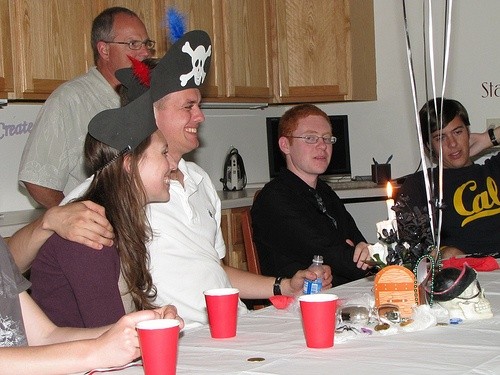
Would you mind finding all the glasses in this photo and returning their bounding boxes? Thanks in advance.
[285,134,337,144]
[102,39,156,50]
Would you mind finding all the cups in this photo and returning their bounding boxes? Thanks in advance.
[372,164,391,186]
[299,293,339,348]
[203,288,240,339]
[135,319,180,375]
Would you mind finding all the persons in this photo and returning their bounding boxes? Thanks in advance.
[10,8,333,332]
[396,96,500,260]
[29,89,175,328]
[251,104,376,288]
[17,7,155,211]
[0,235,183,375]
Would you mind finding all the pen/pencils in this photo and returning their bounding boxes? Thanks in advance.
[386,154,393,164]
[373,157,378,164]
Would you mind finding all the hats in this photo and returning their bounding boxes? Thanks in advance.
[114,29,210,107]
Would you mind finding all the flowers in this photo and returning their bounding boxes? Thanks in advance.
[360,220,411,269]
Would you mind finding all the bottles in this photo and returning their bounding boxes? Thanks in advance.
[303,255,325,296]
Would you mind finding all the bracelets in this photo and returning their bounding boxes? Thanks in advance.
[273,276,285,294]
[440,246,448,251]
[487,128,498,146]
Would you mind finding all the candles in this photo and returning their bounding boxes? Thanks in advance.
[386,182,396,220]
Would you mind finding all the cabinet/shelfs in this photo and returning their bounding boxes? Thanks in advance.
[0,0,377,110]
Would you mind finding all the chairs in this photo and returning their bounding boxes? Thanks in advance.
[240,190,265,310]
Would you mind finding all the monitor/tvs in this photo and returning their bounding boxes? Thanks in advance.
[265,115,352,184]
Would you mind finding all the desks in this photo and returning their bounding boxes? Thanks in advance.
[76,257,500,375]
